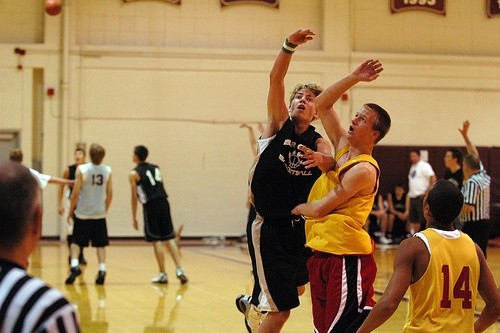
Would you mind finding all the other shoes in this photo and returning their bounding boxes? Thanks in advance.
[65,266,82,284]
[95,270,107,284]
[379,235,392,245]
[176,268,189,284]
[150,272,169,283]
[69,255,87,265]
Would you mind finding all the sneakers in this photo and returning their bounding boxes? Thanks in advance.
[235,294,268,333]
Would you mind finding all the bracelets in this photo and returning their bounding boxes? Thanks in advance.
[282,38,298,54]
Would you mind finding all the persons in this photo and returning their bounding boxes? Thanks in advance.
[0,157,82,333]
[237,30,500,333]
[10,144,187,286]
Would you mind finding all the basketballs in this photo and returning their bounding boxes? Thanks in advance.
[45,0,62,15]
